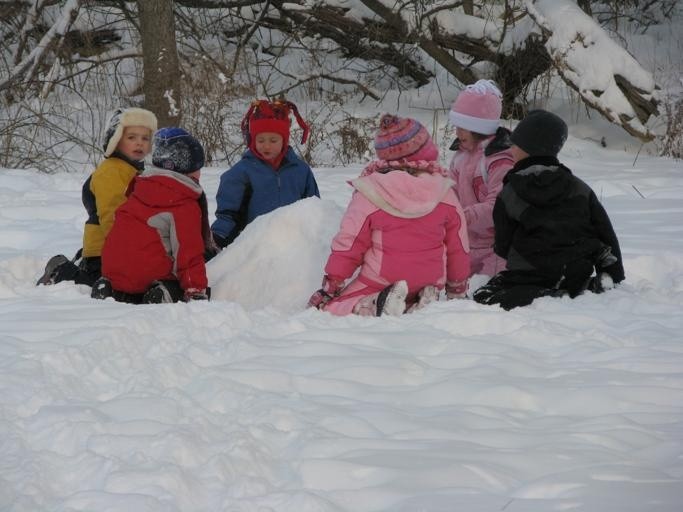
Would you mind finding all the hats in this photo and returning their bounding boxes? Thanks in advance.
[240,100,308,170]
[101,108,158,159]
[151,127,205,173]
[373,112,439,164]
[510,109,569,159]
[449,79,505,137]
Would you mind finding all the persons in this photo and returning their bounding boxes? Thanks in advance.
[34,107,158,288]
[300,113,472,319]
[471,109,626,311]
[88,126,210,306]
[207,97,321,252]
[447,78,520,279]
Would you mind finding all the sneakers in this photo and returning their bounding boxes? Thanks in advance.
[377,280,409,317]
[593,274,614,293]
[413,284,437,310]
[35,254,70,285]
[91,276,113,300]
[70,247,83,266]
[143,286,165,303]
